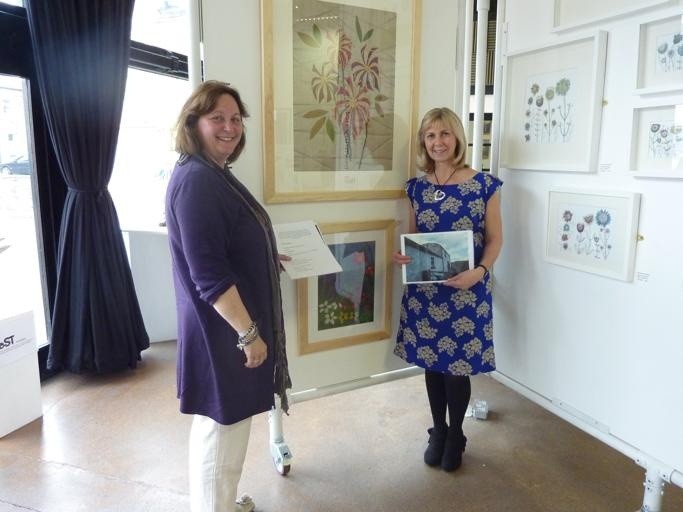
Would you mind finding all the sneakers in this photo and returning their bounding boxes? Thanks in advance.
[236,495,255,512]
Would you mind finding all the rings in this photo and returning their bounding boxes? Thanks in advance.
[432,168,456,201]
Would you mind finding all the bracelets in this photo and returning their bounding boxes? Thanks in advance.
[237,322,256,340]
[236,328,259,351]
[477,263,489,279]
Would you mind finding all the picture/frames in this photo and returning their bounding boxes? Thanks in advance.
[295,220,395,357]
[549,1,680,33]
[628,103,682,180]
[498,28,609,174]
[542,187,641,280]
[630,10,683,98]
[260,1,420,206]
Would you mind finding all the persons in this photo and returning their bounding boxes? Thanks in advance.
[162,79,294,512]
[393,109,505,469]
[338,242,369,324]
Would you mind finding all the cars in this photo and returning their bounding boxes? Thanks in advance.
[0,156,31,176]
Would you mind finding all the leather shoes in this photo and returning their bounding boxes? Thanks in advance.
[424,426,466,471]
